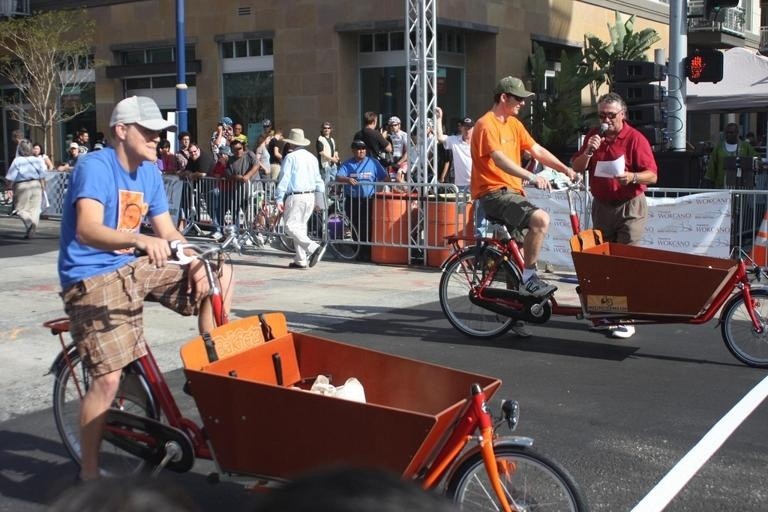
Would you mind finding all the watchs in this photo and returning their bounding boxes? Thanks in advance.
[633,173,637,184]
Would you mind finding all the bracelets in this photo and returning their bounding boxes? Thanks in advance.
[584,150,593,158]
[529,174,536,185]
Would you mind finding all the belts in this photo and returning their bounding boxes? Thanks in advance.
[288,190,317,195]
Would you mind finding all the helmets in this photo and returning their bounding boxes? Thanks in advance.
[387,116,401,128]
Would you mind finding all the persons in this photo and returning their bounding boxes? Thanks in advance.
[273,127,327,269]
[156,117,290,242]
[471,75,576,299]
[316,121,339,196]
[433,105,473,195]
[337,105,433,264]
[572,92,657,339]
[57,95,233,491]
[4,126,107,240]
[699,120,766,188]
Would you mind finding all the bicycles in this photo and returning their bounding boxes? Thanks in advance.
[202,190,323,256]
[439,171,766,367]
[324,185,361,261]
[45,226,587,511]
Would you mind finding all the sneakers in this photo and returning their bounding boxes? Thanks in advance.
[308,244,327,267]
[494,311,533,338]
[610,323,638,339]
[289,262,307,270]
[517,272,559,300]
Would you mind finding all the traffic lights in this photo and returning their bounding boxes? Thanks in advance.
[685,51,721,82]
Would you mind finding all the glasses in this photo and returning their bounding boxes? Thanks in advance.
[189,149,199,153]
[505,93,527,101]
[597,108,624,119]
[494,75,536,101]
[231,147,243,151]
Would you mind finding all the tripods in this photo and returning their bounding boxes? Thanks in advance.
[726,191,768,283]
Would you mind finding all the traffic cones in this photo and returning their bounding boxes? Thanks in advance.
[744,210,768,266]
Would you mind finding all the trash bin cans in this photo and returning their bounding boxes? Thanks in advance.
[427,194,474,267]
[370,192,417,264]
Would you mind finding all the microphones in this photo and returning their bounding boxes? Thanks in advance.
[586,122,610,154]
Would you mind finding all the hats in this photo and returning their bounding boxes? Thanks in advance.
[108,94,178,136]
[460,116,474,127]
[68,142,79,149]
[351,138,367,150]
[217,122,229,130]
[281,128,311,147]
[94,142,104,150]
